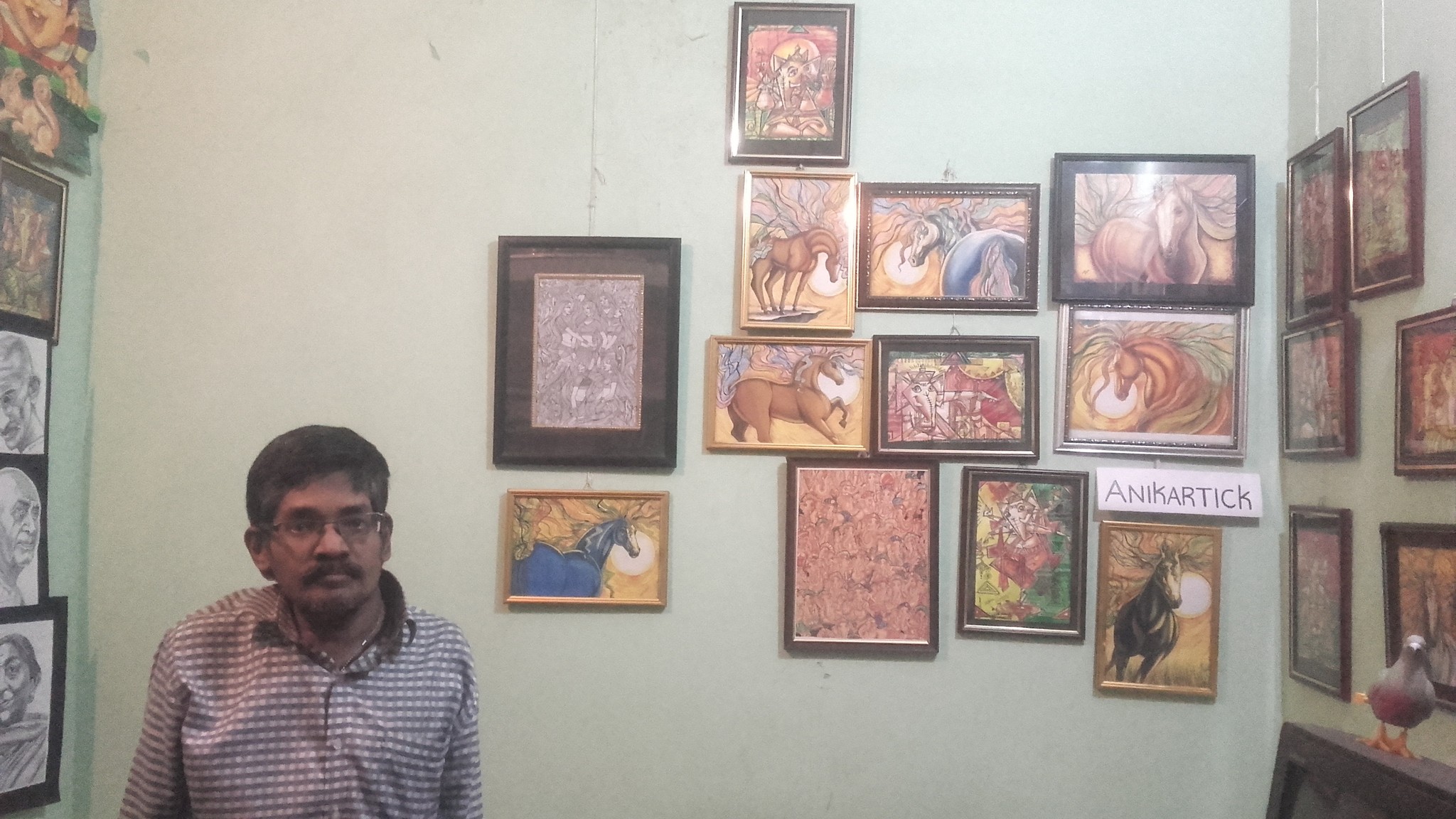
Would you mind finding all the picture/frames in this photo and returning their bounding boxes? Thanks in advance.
[1,153,72,810]
[471,2,1456,723]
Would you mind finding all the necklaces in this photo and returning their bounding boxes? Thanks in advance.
[341,605,386,671]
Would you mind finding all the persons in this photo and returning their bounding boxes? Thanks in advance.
[115,425,483,819]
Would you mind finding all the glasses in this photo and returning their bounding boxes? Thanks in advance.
[255,513,384,543]
